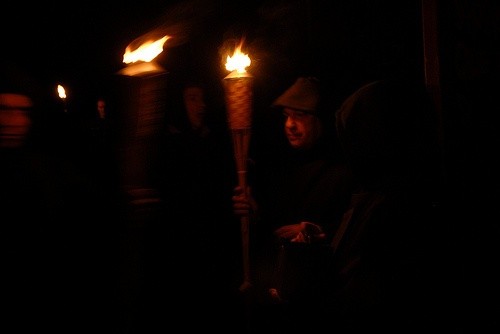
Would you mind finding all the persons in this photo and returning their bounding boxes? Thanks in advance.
[1,70,399,334]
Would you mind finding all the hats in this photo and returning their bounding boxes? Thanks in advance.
[272,76,333,117]
